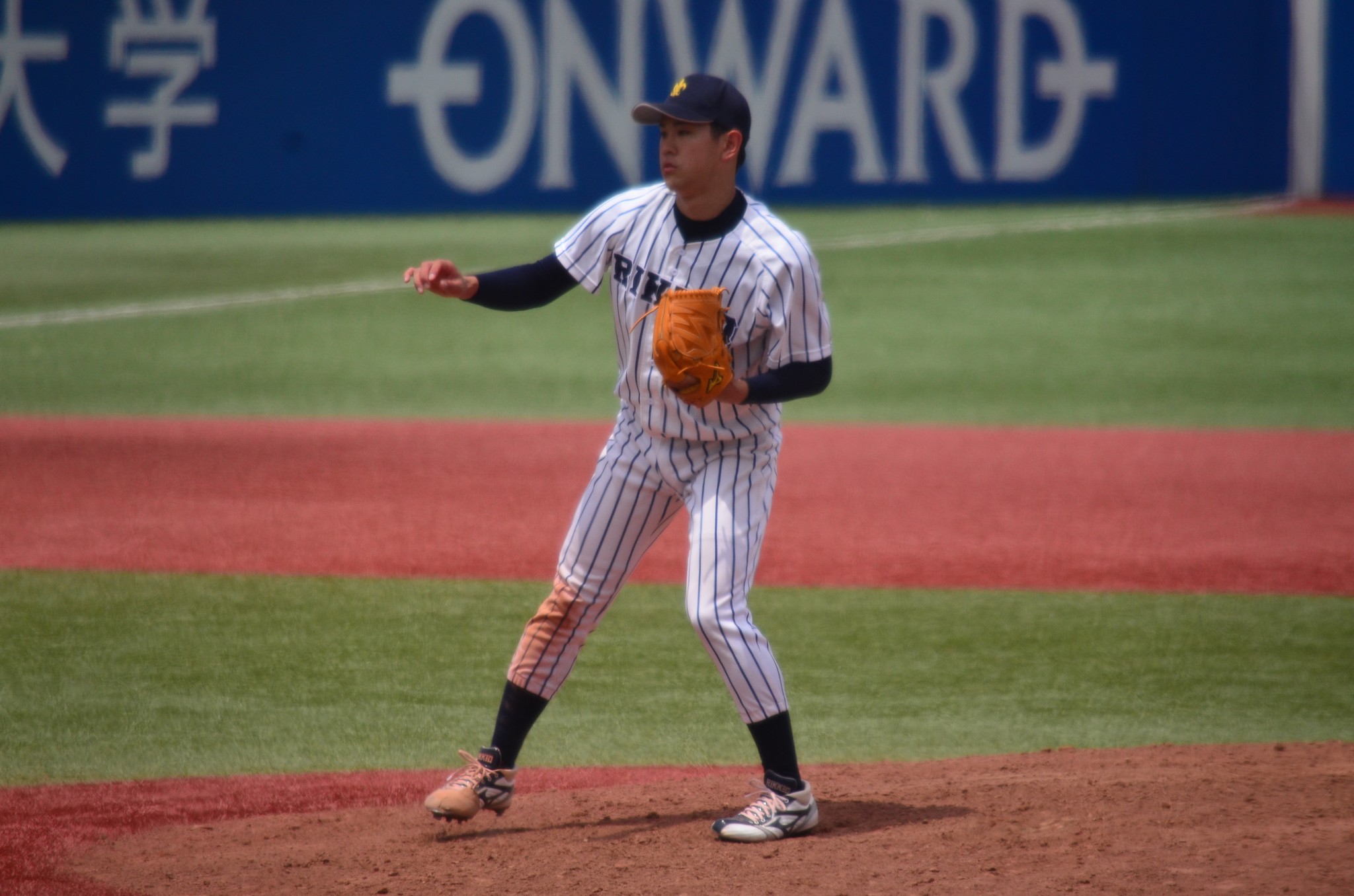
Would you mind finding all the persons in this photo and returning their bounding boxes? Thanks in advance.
[408,74,834,844]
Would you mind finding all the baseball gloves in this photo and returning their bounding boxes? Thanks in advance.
[652,288,734,409]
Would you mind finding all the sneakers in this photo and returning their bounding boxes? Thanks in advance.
[424,746,518,823]
[711,770,819,842]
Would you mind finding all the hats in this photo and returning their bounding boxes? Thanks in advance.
[631,74,751,147]
